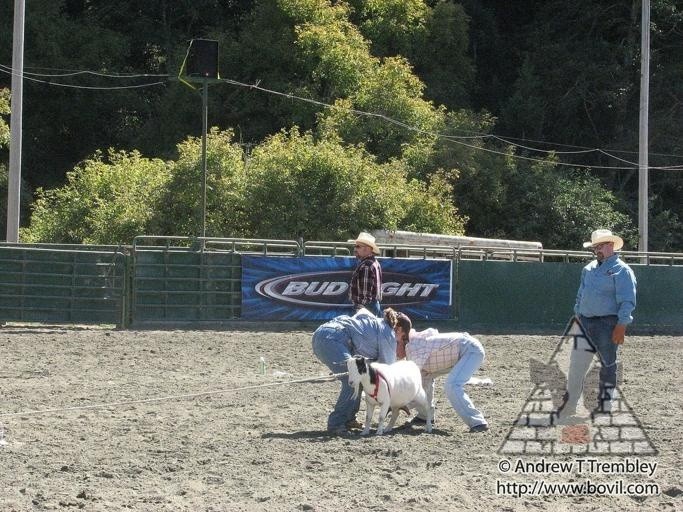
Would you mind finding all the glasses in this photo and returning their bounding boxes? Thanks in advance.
[588,244,607,250]
[353,245,368,248]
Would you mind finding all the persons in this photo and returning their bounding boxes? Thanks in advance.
[306,304,413,436]
[342,230,387,319]
[396,326,489,433]
[547,228,638,421]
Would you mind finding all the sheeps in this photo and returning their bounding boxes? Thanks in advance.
[333,352,432,436]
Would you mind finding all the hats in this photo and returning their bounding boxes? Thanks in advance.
[349,232,380,254]
[583,230,623,250]
[397,312,410,342]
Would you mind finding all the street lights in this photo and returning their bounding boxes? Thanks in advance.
[184,38,221,253]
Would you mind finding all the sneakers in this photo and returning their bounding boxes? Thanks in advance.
[328,420,362,434]
[470,424,487,432]
[412,417,434,423]
[591,406,610,418]
[559,402,575,410]
